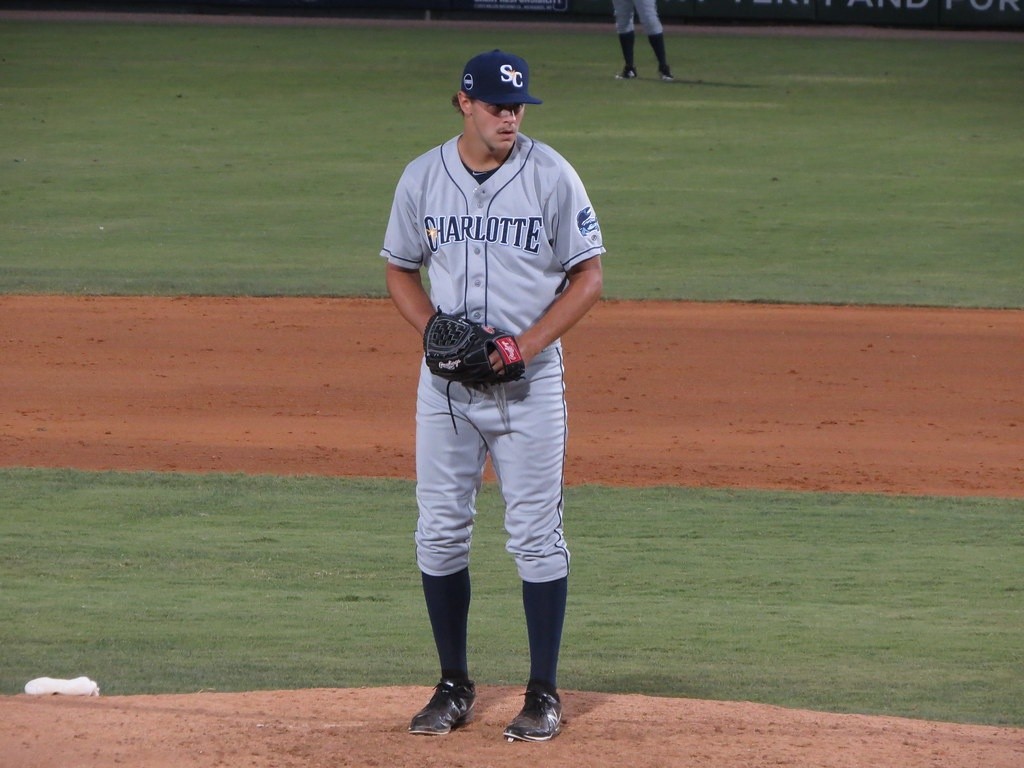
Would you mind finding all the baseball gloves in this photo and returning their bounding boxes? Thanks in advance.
[421,304,529,435]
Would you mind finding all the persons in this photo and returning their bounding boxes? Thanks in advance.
[378,47,608,744]
[609,0,676,81]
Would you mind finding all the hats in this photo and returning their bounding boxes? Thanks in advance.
[462,49,545,105]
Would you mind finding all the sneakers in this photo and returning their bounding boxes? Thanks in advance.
[658,64,674,80]
[409,677,476,735]
[503,689,563,742]
[616,66,638,79]
